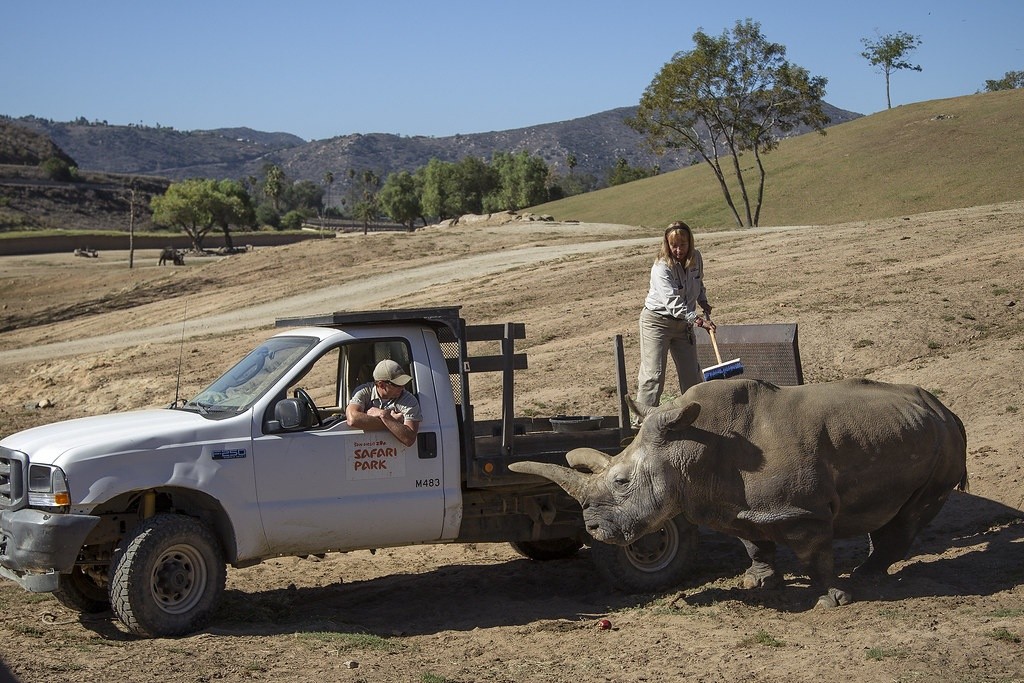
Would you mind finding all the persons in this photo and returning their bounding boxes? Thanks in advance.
[345,358,424,447]
[632,220,717,428]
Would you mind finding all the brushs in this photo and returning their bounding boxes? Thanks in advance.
[702,311,744,382]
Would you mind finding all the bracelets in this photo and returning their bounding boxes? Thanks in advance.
[698,319,703,327]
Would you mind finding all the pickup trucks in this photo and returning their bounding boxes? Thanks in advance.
[0,300,805,641]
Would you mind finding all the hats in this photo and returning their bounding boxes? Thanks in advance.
[373,359,414,385]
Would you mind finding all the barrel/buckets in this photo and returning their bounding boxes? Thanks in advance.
[549,414,604,432]
